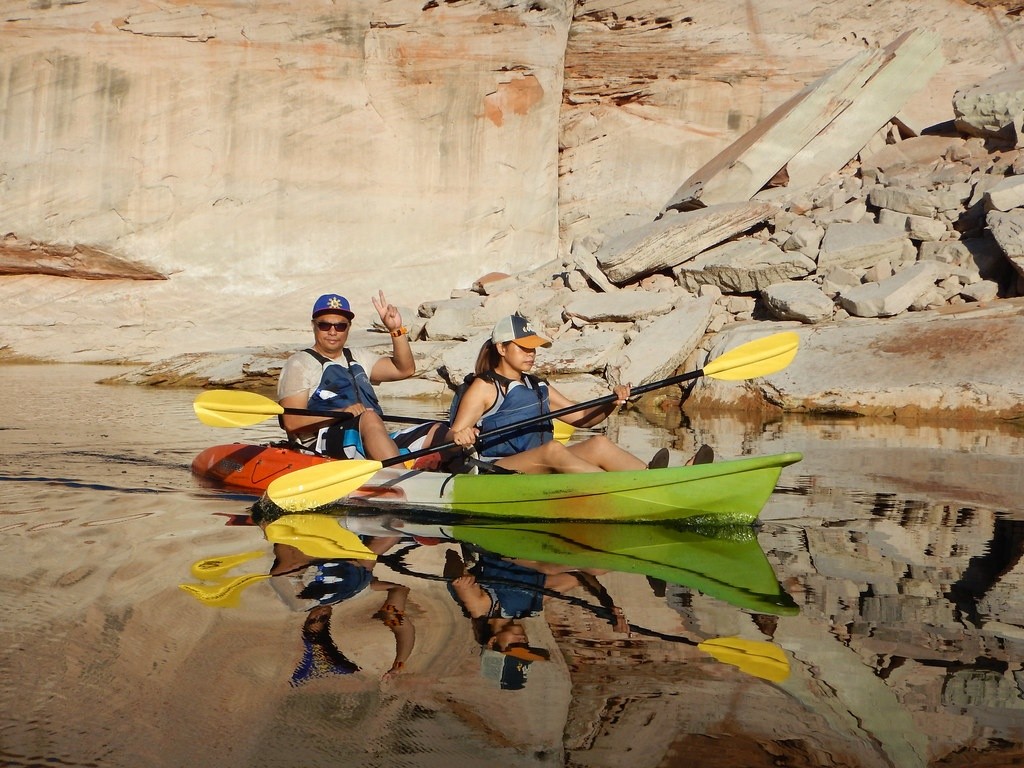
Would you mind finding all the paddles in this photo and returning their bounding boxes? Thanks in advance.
[265,331,800,516]
[262,511,791,686]
[190,387,573,447]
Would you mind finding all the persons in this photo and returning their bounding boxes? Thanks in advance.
[445,316,713,474]
[278,290,448,470]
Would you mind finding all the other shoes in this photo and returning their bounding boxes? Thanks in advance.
[646,448,669,469]
[685,444,714,466]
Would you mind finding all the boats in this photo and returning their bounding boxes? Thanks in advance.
[201,487,804,618]
[189,441,803,523]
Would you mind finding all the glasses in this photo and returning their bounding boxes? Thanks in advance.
[315,320,347,332]
[308,615,328,624]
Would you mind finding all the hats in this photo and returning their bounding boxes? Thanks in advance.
[491,315,552,349]
[312,294,355,319]
[479,643,546,689]
[302,630,330,644]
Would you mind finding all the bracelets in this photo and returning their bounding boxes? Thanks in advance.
[390,327,407,337]
